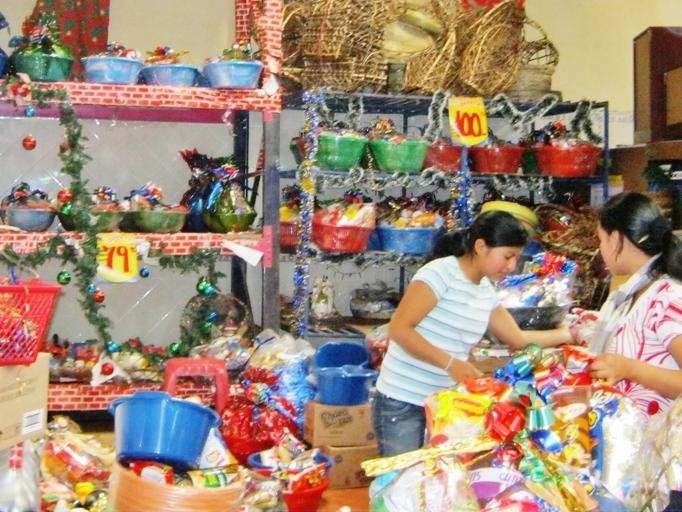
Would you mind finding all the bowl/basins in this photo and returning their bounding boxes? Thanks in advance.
[288,138,603,179]
[246,448,333,511]
[314,223,437,253]
[108,387,222,469]
[2,205,259,234]
[311,339,379,406]
[503,307,572,332]
[2,55,263,93]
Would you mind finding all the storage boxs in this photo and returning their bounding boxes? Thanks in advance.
[304,402,374,452]
[316,446,376,493]
[0,353,51,446]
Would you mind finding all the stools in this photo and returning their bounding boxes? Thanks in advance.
[163,359,231,414]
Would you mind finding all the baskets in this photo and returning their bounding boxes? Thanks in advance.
[0,279,62,365]
[535,201,607,308]
[280,1,557,98]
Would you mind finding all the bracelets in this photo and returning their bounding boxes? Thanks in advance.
[443,356,455,371]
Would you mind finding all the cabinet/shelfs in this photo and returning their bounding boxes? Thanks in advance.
[0,0,278,419]
[278,90,609,345]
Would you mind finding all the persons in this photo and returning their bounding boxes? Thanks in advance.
[371,209,576,462]
[581,190,681,512]
